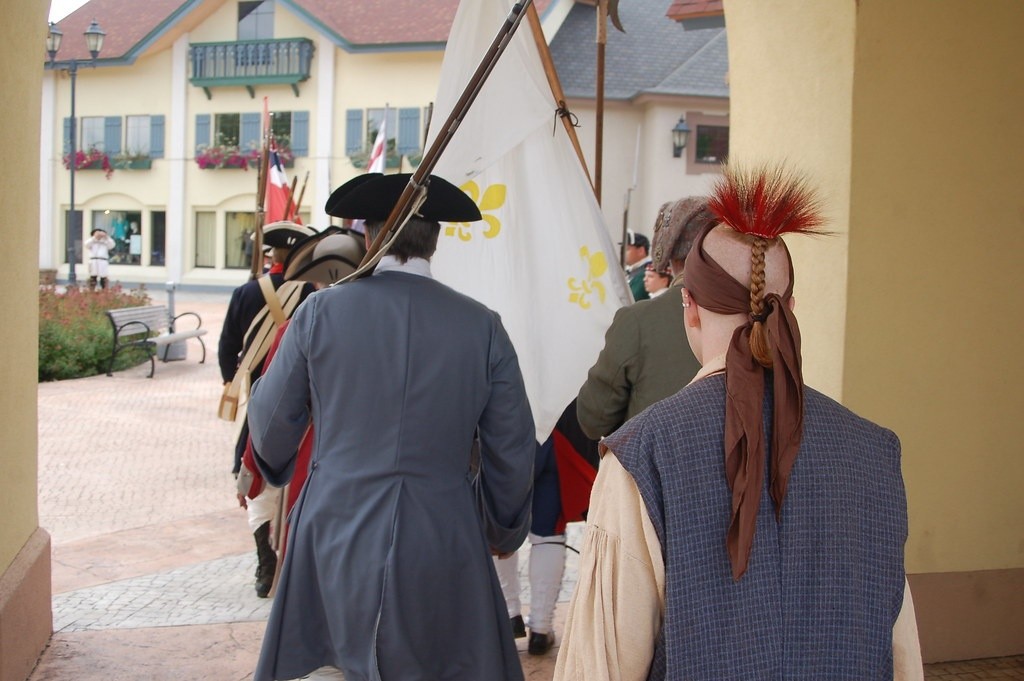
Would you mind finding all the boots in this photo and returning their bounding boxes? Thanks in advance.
[253,520,279,598]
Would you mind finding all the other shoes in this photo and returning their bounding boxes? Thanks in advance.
[529,631,554,655]
[509,615,526,638]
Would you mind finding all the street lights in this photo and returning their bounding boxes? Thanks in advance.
[46,17,109,295]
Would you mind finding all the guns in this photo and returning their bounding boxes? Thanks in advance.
[423,100,434,156]
[283,170,310,221]
[619,185,632,270]
[352,0,536,278]
[249,111,274,280]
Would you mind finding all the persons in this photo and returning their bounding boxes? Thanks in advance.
[552,160,925,681]
[237,228,249,267]
[219,173,565,681]
[85,229,116,292]
[109,211,129,263]
[125,222,141,264]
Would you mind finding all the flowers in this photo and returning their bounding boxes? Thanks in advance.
[195,132,248,169]
[64,144,114,179]
[250,131,297,162]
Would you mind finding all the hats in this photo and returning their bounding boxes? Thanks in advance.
[325,173,482,222]
[250,219,320,247]
[283,223,378,289]
[91,228,106,235]
[651,196,719,271]
[618,231,648,246]
[645,259,673,277]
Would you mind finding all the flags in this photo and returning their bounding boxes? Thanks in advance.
[350,118,386,233]
[423,0,637,536]
[263,130,303,227]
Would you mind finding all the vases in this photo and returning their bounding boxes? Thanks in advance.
[247,160,292,169]
[204,159,245,168]
[85,160,110,169]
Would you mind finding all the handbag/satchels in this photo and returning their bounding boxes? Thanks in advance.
[217,380,238,422]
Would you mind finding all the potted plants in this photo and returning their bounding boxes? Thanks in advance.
[112,152,152,169]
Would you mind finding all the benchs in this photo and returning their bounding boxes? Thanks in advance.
[106,305,208,378]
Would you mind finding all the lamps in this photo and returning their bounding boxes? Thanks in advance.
[670,115,692,158]
